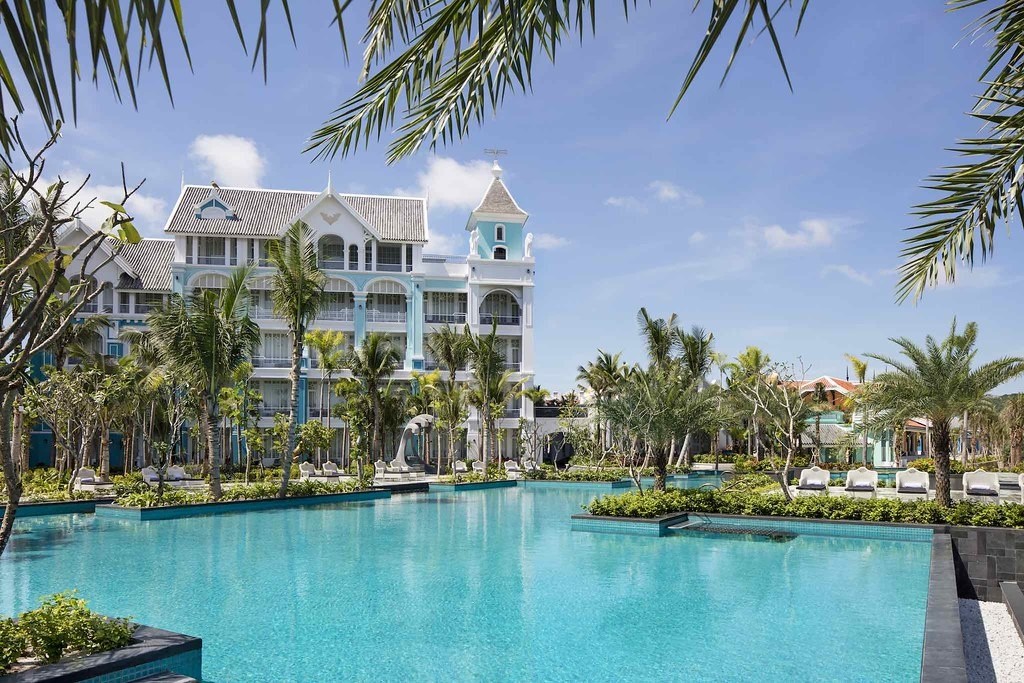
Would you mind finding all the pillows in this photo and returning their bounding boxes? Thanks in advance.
[332,470,338,474]
[80,478,95,482]
[149,476,158,479]
[853,481,871,488]
[308,471,315,475]
[905,482,921,489]
[806,479,821,486]
[175,474,183,478]
[970,483,991,489]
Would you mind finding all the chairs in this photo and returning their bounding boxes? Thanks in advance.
[299,461,329,483]
[962,469,1001,504]
[845,467,878,498]
[322,461,350,484]
[168,465,191,480]
[374,459,410,482]
[523,460,540,472]
[72,467,115,496]
[504,460,521,473]
[452,460,468,472]
[390,459,425,481]
[142,466,160,485]
[472,459,484,472]
[896,468,929,498]
[795,466,831,496]
